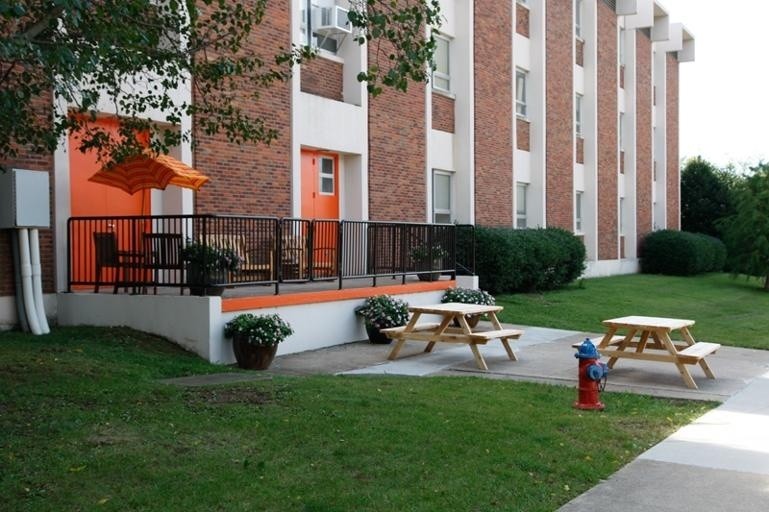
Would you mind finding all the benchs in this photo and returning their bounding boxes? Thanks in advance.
[676,341,721,366]
[379,322,442,340]
[467,328,525,345]
[572,335,633,353]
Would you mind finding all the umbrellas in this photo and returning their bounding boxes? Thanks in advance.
[87,146,212,253]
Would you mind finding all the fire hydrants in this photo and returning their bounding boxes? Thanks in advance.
[573,337,607,410]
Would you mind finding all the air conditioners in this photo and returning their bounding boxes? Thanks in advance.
[314,5,354,36]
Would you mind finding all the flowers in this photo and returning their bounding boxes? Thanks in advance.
[441,286,495,319]
[224,313,293,347]
[406,241,450,263]
[354,293,410,330]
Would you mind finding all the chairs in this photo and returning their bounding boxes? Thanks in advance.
[93,231,336,294]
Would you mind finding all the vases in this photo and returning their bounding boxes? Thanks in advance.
[232,334,279,370]
[365,320,393,344]
[414,256,443,281]
[453,313,481,327]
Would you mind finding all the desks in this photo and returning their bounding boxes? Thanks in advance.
[596,315,716,389]
[387,302,518,370]
